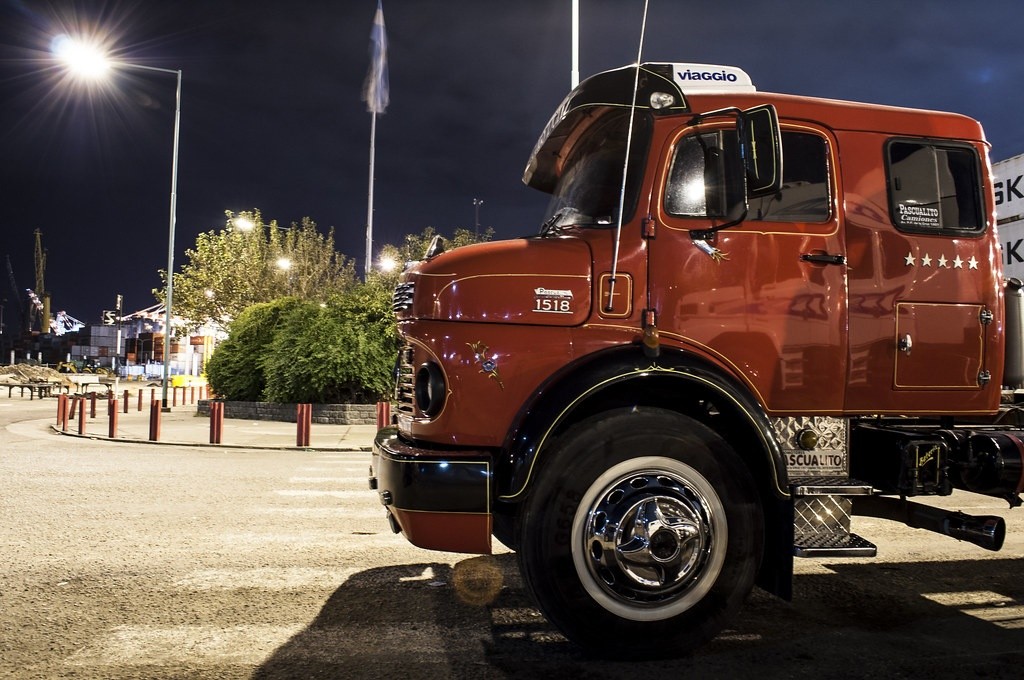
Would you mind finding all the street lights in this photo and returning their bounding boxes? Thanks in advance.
[51,38,182,412]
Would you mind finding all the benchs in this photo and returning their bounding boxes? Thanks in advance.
[81,381,112,393]
[39,383,70,394]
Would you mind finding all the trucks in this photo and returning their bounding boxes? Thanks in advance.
[372,59,1023,670]
